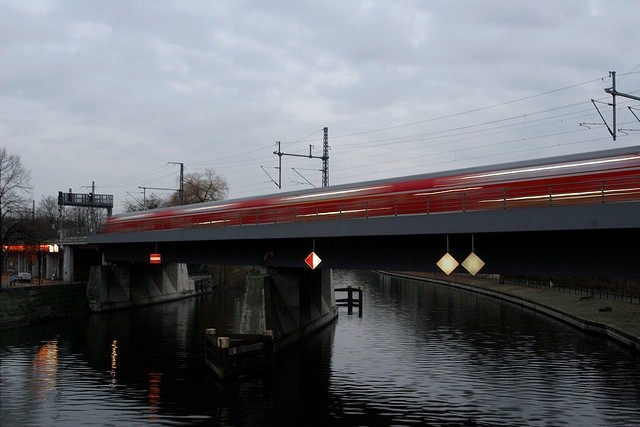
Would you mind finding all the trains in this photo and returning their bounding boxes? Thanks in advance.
[100,145,640,233]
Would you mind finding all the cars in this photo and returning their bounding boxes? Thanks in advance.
[10,271,32,282]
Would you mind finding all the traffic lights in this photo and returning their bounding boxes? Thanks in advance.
[150,254,161,263]
[306,251,322,269]
[437,252,459,276]
[462,253,485,275]
